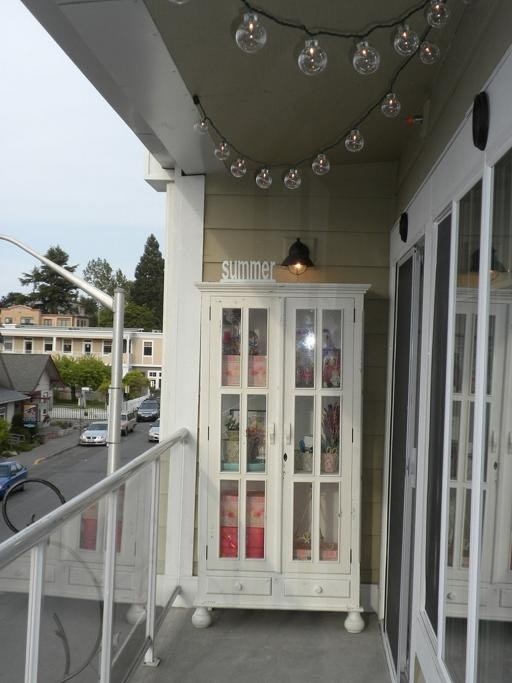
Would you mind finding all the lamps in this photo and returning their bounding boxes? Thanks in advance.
[174,3,450,196]
[280,238,313,278]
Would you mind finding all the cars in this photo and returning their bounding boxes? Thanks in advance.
[0,460,28,500]
[80,398,160,446]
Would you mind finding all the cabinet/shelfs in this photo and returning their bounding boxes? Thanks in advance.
[189,281,375,633]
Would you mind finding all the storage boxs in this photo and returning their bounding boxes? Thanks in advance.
[217,492,263,557]
[220,352,267,388]
